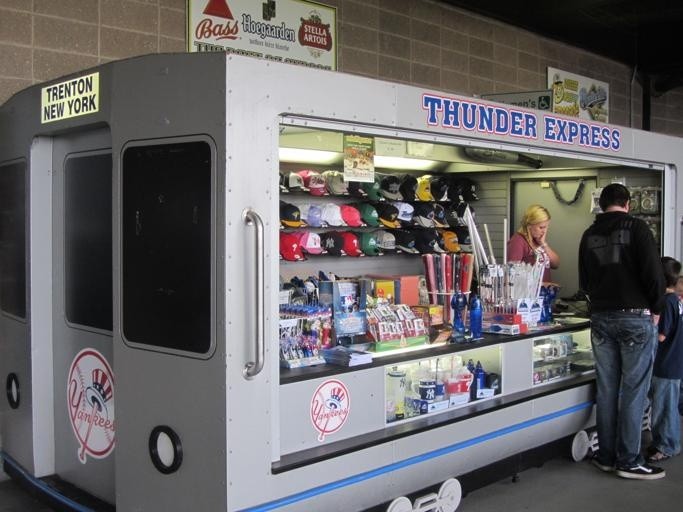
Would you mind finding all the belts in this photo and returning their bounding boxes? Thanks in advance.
[622,308,653,316]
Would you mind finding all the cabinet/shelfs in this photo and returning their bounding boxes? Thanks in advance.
[280,187,480,265]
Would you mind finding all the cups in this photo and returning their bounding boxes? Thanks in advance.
[386,370,410,421]
[409,378,437,404]
[533,334,576,386]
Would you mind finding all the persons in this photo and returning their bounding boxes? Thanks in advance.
[576,183,667,481]
[506,203,561,289]
[644,254,682,463]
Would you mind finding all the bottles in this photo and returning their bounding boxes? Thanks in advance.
[473,360,487,401]
[451,289,468,341]
[466,359,477,400]
[470,295,482,341]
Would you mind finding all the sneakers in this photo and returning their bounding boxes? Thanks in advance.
[591,446,669,479]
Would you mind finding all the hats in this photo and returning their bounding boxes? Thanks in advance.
[280,171,480,261]
